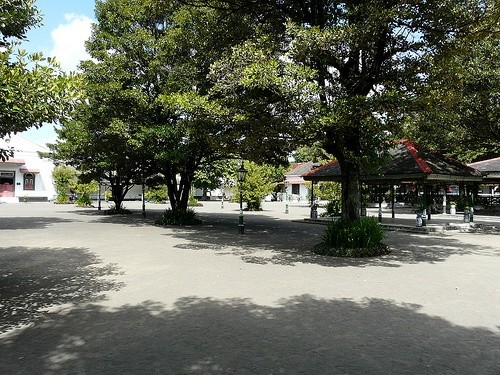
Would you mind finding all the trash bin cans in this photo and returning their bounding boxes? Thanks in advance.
[464,209,470,224]
[361,201,367,217]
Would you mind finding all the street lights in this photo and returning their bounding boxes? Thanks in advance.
[236,160,249,234]
